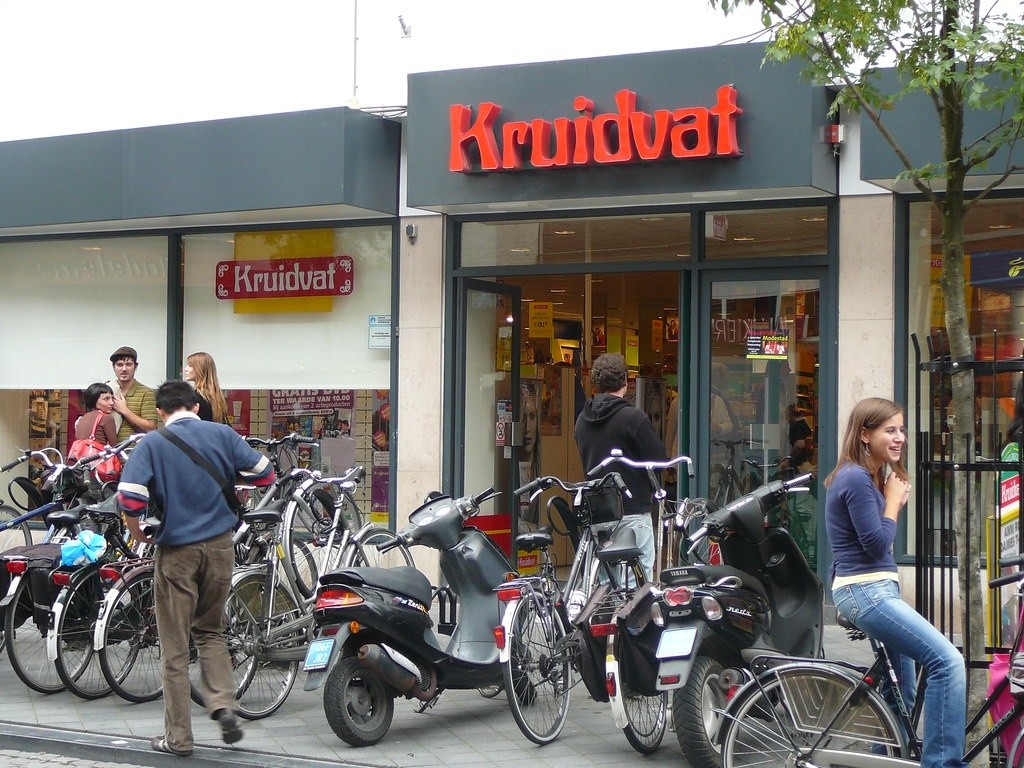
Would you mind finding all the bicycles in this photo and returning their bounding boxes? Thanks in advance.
[491,471,633,745]
[0,434,417,721]
[710,438,811,559]
[583,447,709,758]
[709,551,1024,768]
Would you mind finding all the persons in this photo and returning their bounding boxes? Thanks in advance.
[662,362,748,514]
[287,421,300,434]
[592,327,605,346]
[825,397,967,768]
[116,381,274,758]
[185,352,228,428]
[781,405,814,476]
[666,320,679,340]
[645,387,665,445]
[75,383,120,541]
[319,417,350,438]
[516,384,541,523]
[74,346,158,471]
[574,353,668,599]
[764,340,785,354]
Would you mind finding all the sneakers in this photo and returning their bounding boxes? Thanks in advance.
[219,709,243,744]
[151,735,194,756]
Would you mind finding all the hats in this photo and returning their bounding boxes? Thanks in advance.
[110,347,137,362]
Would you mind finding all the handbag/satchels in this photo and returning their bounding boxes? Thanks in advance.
[30,544,105,626]
[0,545,33,631]
[571,583,610,703]
[67,412,121,481]
[618,581,666,697]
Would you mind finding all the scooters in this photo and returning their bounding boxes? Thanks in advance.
[649,470,826,768]
[303,485,538,747]
